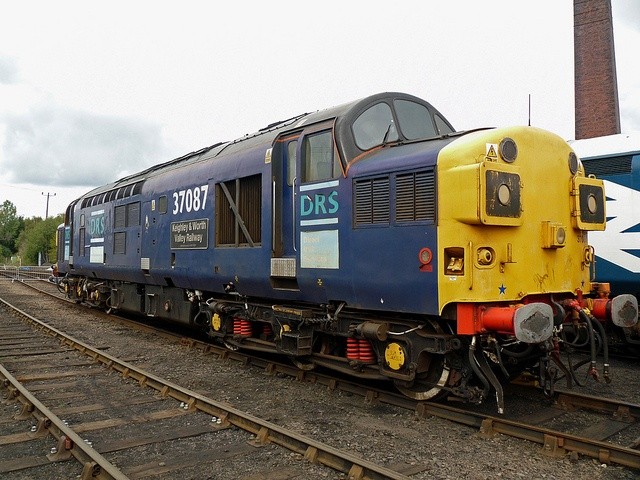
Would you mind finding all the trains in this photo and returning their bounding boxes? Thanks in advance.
[51,91,638,416]
[563,134,639,354]
